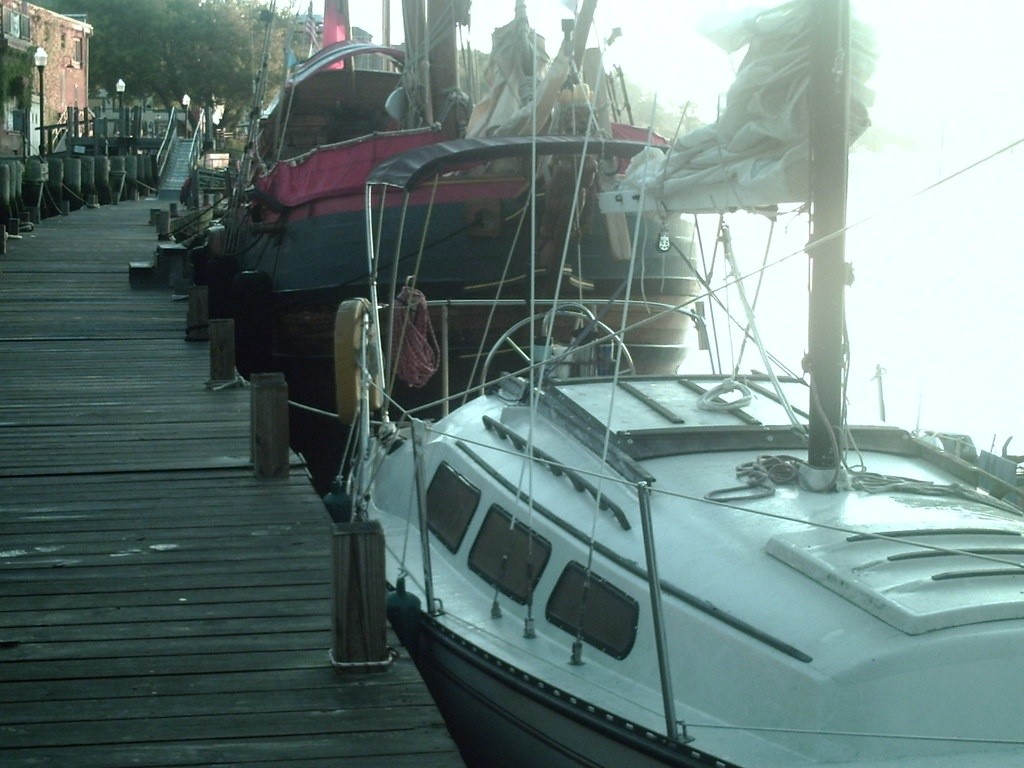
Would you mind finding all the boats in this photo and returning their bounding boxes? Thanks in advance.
[206,0,697,457]
[333,132,1022,767]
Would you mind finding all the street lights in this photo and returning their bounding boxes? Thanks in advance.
[116,78,125,139]
[33,47,48,158]
[183,94,190,138]
